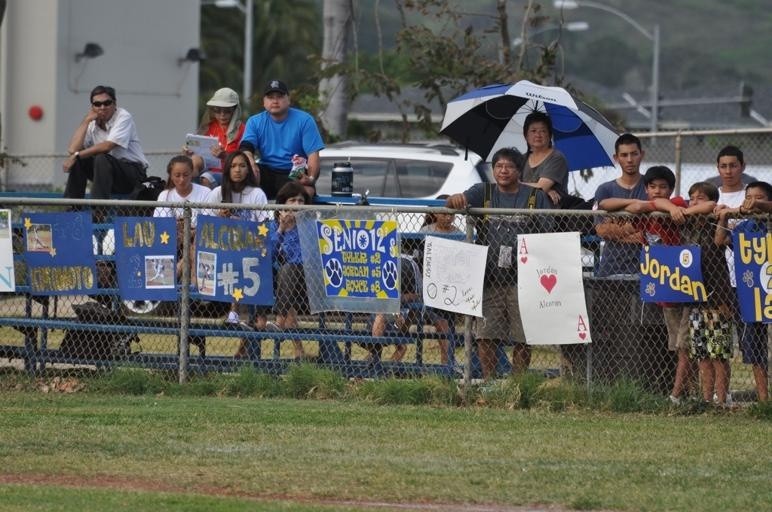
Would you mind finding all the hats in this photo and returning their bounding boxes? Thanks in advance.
[206,88,239,107]
[263,81,289,95]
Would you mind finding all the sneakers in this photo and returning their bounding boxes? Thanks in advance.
[238,320,257,331]
[266,321,283,333]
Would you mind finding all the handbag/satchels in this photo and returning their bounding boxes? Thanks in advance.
[554,187,596,234]
[118,176,166,217]
[71,302,132,326]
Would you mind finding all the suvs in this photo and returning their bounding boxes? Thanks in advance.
[87,145,485,320]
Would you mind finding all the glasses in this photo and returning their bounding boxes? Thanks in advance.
[212,107,234,114]
[92,100,113,107]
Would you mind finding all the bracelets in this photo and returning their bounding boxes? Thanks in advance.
[74,151,81,160]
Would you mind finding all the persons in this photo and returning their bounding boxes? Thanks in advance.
[64,86,149,222]
[372,111,570,387]
[153,78,325,360]
[593,134,771,412]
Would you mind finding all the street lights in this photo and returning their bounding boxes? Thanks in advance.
[553,0,661,148]
[211,0,256,124]
[499,21,591,66]
